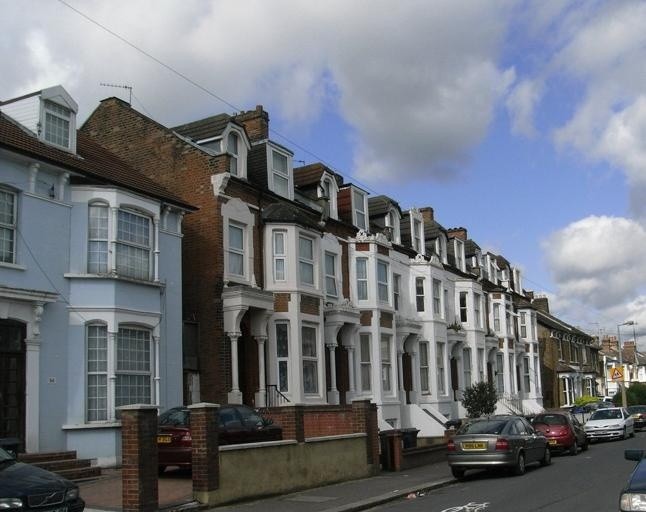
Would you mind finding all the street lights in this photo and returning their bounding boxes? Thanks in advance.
[616,321,635,409]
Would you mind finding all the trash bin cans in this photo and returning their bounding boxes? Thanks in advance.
[378,428,420,472]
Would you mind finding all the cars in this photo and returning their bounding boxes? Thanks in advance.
[157,404,282,475]
[0,436,84,512]
[447,404,646,476]
[619,449,646,511]
